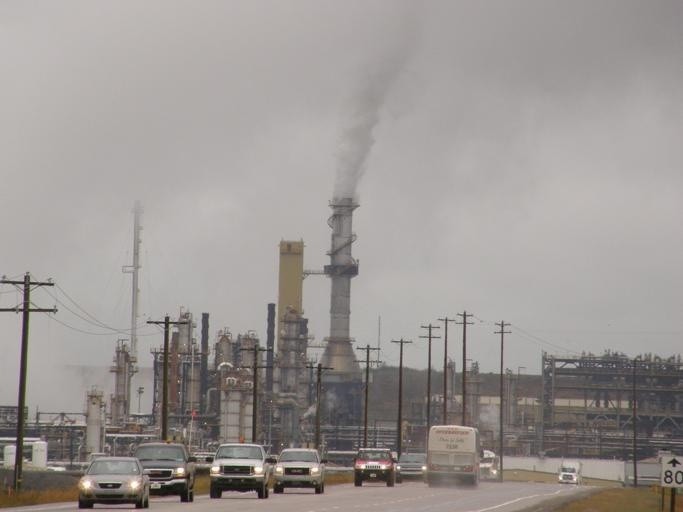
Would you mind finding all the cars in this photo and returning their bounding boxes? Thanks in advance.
[76,454,150,509]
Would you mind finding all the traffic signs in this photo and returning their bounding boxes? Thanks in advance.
[660,455,683,488]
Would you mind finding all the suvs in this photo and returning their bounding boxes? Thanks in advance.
[271,447,326,495]
[351,424,503,489]
[206,443,276,500]
[135,439,196,503]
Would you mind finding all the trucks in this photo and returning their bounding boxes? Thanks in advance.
[557,460,581,485]
